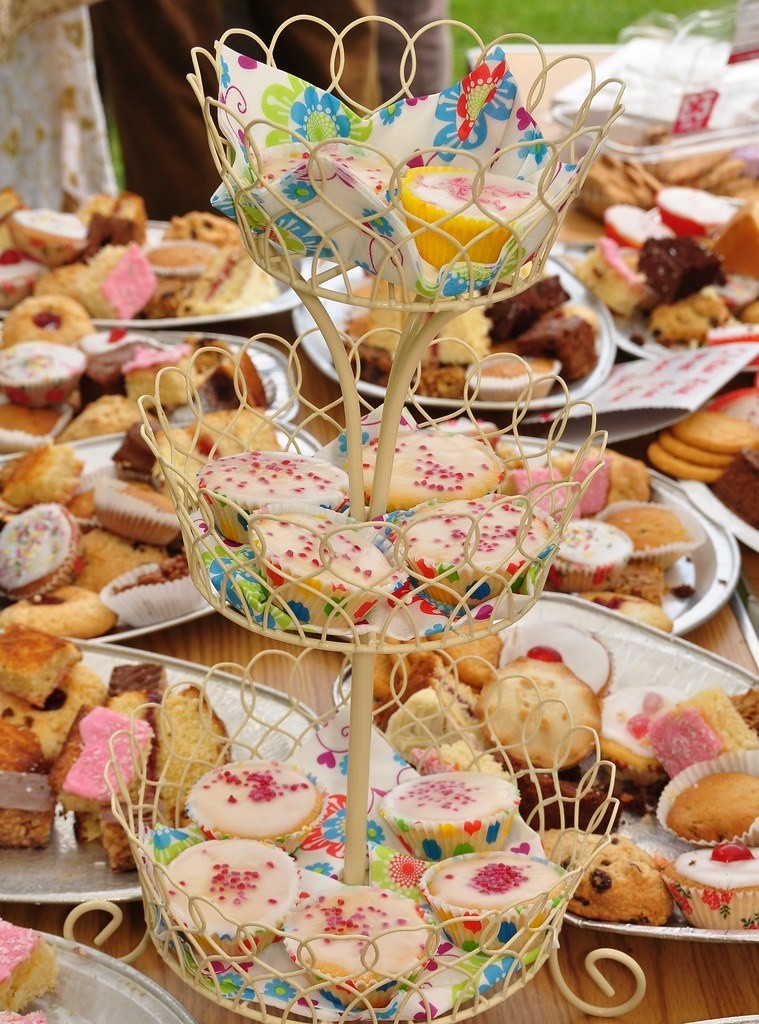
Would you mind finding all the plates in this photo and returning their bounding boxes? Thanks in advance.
[0,41,759,1024]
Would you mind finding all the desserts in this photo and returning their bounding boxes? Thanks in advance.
[0,128,759,1024]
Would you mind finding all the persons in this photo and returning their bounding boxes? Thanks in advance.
[0,0,454,219]
